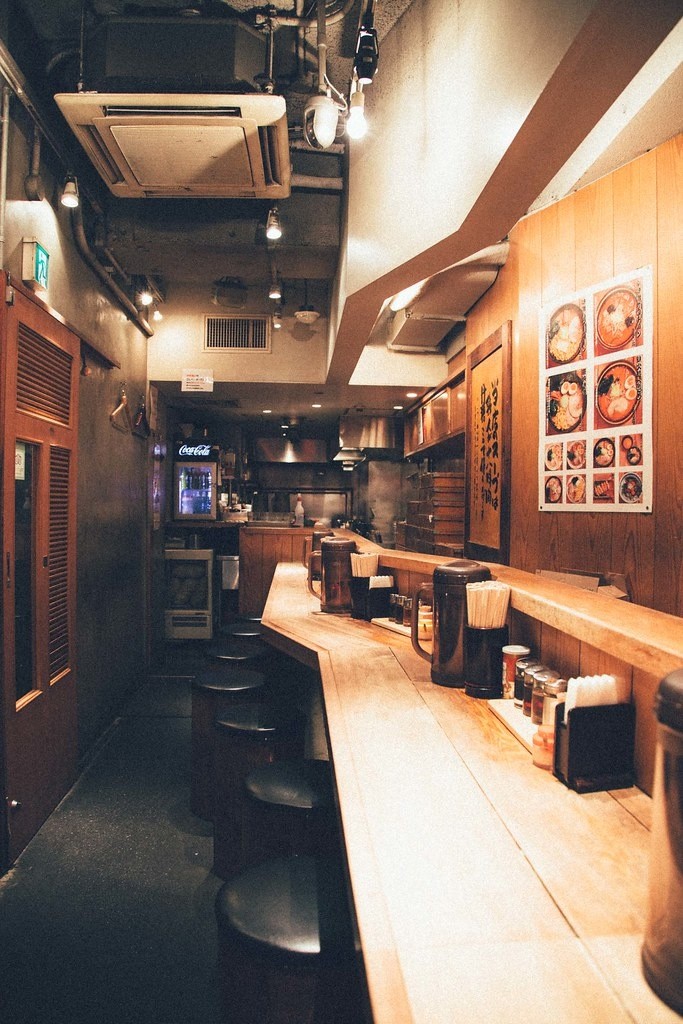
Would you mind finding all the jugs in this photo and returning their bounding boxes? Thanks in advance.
[641,668,683,1015]
[301,530,336,577]
[411,559,498,688]
[307,537,360,614]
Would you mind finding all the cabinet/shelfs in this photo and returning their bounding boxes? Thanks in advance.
[402,369,465,462]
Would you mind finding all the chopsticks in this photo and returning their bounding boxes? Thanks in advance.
[466,580,510,628]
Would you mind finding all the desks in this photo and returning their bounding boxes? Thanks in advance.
[255,531,683,1024]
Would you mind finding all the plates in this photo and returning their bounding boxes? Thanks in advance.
[593,480,614,498]
[566,475,586,503]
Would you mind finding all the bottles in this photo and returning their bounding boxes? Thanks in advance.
[181,469,211,489]
[533,726,555,771]
[389,593,434,633]
[182,491,211,514]
[502,645,567,725]
[295,493,304,527]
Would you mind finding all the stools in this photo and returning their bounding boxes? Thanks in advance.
[194,614,367,1024]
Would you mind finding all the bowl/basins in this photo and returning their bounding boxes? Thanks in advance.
[546,444,563,471]
[619,473,642,503]
[594,438,614,467]
[595,361,641,424]
[545,476,562,503]
[596,286,641,349]
[627,446,641,465]
[567,441,585,469]
[548,374,587,433]
[621,435,633,450]
[548,303,585,364]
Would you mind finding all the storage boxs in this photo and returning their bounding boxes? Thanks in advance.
[535,565,632,603]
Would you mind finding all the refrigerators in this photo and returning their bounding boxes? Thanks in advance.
[173,444,219,520]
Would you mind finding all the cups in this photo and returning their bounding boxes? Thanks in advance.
[464,623,509,699]
[350,577,369,619]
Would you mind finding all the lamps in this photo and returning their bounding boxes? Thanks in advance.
[273,306,282,328]
[265,204,282,239]
[60,170,80,208]
[293,279,321,325]
[353,0,381,86]
[269,284,282,299]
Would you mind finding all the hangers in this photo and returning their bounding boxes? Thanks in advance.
[111,382,134,434]
[134,393,151,440]
